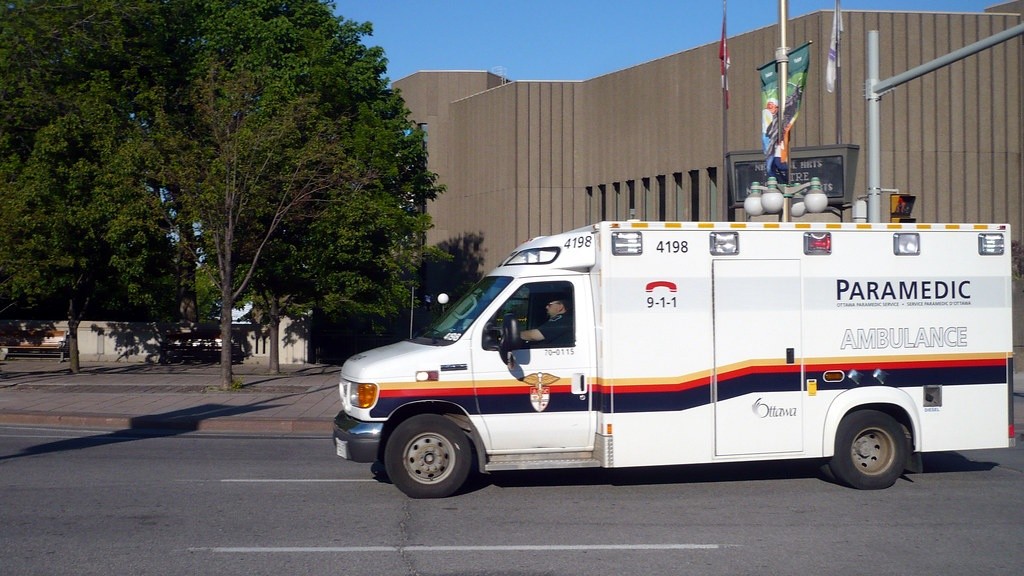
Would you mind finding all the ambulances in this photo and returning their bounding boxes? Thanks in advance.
[332,218,1019,499]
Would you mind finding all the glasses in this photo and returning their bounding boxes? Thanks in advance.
[547,302,561,307]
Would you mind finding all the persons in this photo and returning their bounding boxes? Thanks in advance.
[499,298,573,347]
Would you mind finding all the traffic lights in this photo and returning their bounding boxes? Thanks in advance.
[889,192,918,223]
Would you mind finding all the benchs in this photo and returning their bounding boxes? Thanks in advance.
[0,330,69,362]
[161,329,242,365]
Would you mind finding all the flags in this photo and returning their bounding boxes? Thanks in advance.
[826,0,844,94]
[718,15,732,109]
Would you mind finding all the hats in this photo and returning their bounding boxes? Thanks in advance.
[544,292,564,302]
